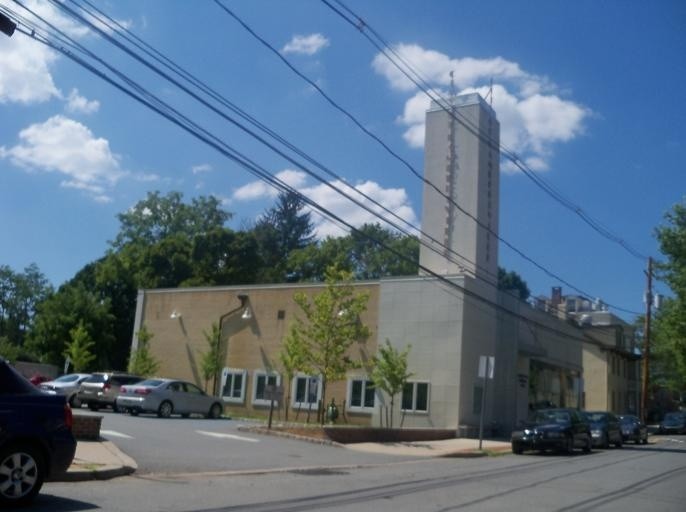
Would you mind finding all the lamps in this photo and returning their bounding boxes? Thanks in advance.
[240,304,253,320]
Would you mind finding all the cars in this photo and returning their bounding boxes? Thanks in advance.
[76,371,147,413]
[615,414,648,447]
[580,409,623,450]
[37,371,90,408]
[510,408,591,456]
[0,356,76,510]
[21,373,53,387]
[659,412,685,435]
[115,376,225,420]
